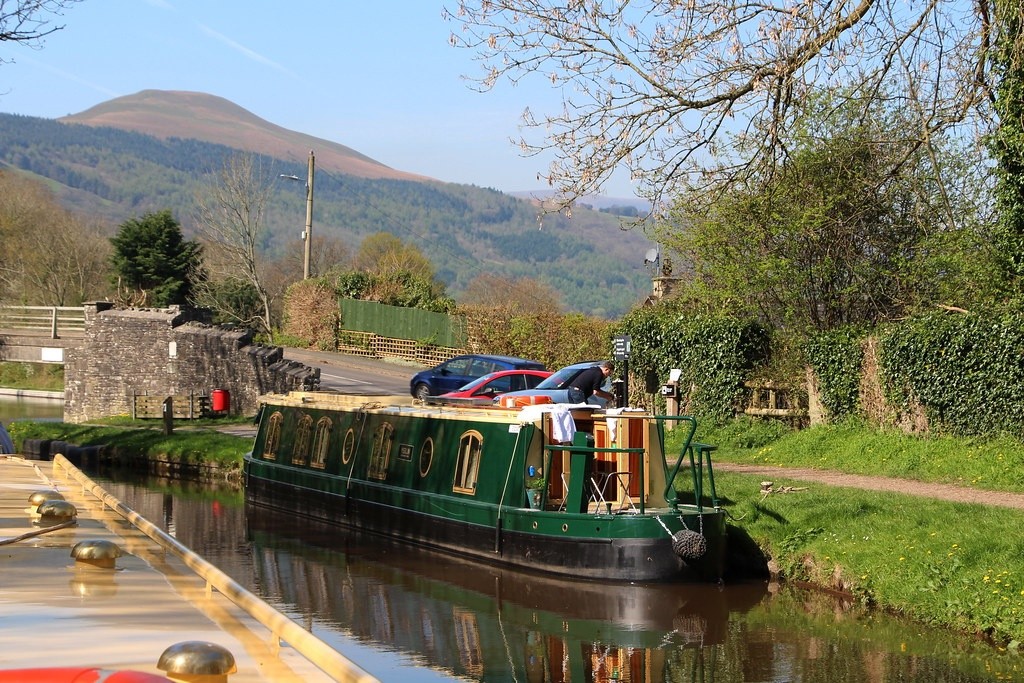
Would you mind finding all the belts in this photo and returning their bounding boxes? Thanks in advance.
[569,385,581,391]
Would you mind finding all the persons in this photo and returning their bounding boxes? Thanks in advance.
[568,361,614,406]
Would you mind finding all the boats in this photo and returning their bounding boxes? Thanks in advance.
[239,503,731,683]
[241,395,729,585]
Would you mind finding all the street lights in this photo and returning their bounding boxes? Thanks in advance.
[279,173,314,283]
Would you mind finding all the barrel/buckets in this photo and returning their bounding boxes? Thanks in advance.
[212,389,230,411]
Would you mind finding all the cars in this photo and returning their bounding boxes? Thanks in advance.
[410,353,548,409]
[439,370,558,407]
[492,360,612,412]
